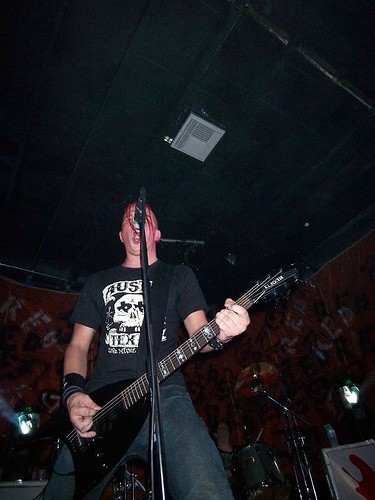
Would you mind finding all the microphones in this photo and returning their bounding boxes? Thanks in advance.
[134,187,146,229]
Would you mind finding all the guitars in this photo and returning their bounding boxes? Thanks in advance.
[38,261,303,493]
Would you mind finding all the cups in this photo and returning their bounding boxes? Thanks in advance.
[32,470,46,482]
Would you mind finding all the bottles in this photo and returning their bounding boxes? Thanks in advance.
[324,424,339,447]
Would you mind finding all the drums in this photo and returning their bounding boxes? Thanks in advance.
[232,440,288,496]
